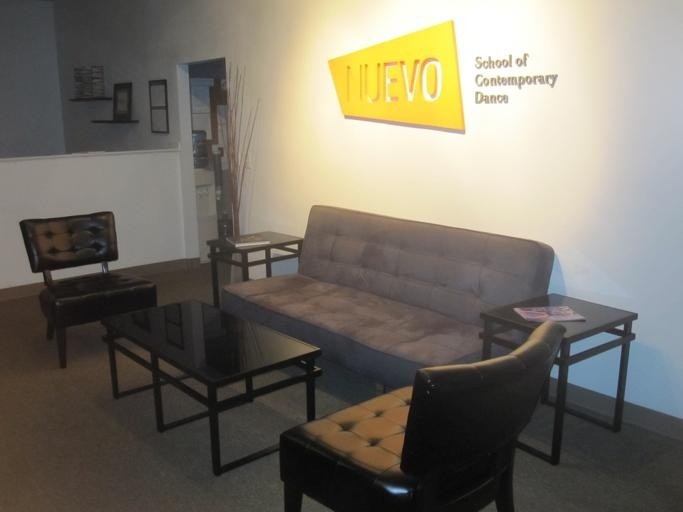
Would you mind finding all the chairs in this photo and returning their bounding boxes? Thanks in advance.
[280,320,567,512]
[20,211,158,369]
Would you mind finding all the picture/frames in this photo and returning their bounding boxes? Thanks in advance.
[113,82,132,120]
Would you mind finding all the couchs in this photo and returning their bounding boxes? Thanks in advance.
[218,204,555,389]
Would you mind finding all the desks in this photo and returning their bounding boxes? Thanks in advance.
[206,232,303,307]
[478,293,637,465]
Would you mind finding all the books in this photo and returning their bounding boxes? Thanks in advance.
[513,305,587,322]
[226,233,271,247]
